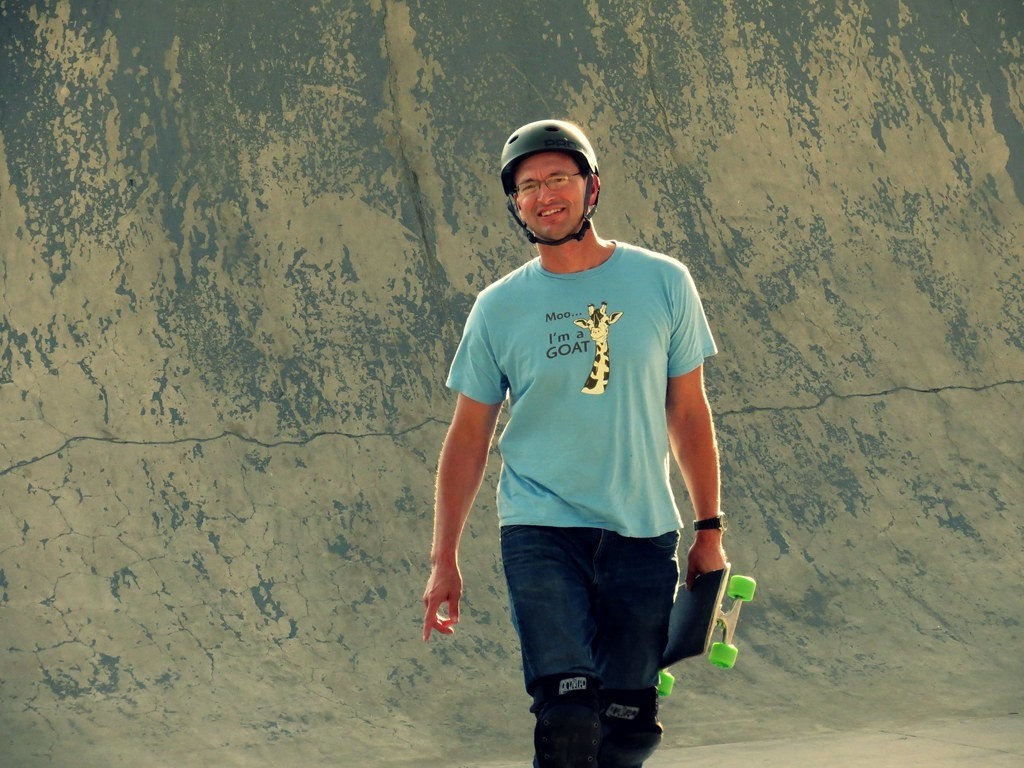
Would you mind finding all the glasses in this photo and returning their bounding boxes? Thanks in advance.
[513,171,589,196]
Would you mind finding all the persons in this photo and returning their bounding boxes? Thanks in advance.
[423,120,727,768]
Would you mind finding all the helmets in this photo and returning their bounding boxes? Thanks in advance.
[500,119,598,196]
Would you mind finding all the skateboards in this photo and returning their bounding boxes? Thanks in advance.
[656,564,756,694]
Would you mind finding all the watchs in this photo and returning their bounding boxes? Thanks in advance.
[693,512,728,532]
